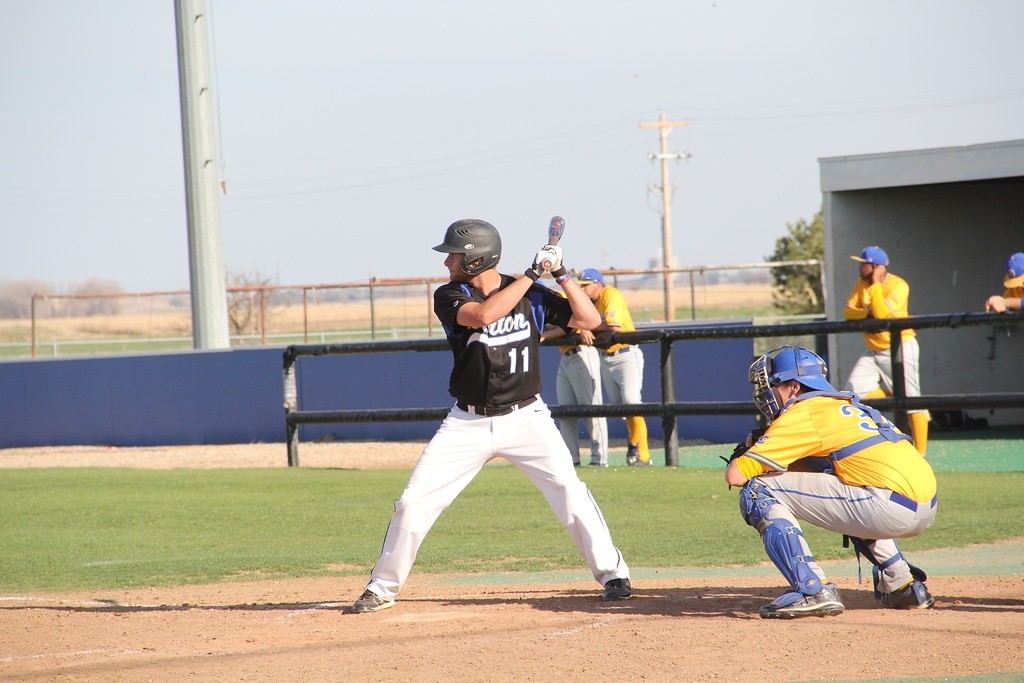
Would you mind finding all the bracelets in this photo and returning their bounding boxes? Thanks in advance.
[556,274,570,286]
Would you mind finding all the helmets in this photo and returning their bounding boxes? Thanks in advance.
[771,348,836,392]
[849,246,889,266]
[431,218,501,276]
[1003,252,1024,288]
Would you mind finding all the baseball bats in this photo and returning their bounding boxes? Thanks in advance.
[541,216,565,269]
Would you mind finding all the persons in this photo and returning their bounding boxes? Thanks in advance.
[539,267,650,467]
[842,245,932,456]
[719,344,938,619]
[984,252,1024,314]
[348,218,632,614]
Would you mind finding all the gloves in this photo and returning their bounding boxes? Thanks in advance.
[543,244,569,285]
[525,249,555,281]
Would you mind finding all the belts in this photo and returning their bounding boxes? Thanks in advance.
[456,396,537,416]
[565,347,582,355]
[603,348,629,357]
[889,491,937,512]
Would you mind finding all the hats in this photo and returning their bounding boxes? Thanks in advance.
[574,269,604,284]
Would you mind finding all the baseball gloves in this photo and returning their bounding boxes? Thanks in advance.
[728,425,770,464]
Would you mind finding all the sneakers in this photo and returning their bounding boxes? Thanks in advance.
[603,576,631,601]
[626,437,639,465]
[875,581,935,608]
[634,459,652,466]
[759,582,844,619]
[351,586,394,614]
